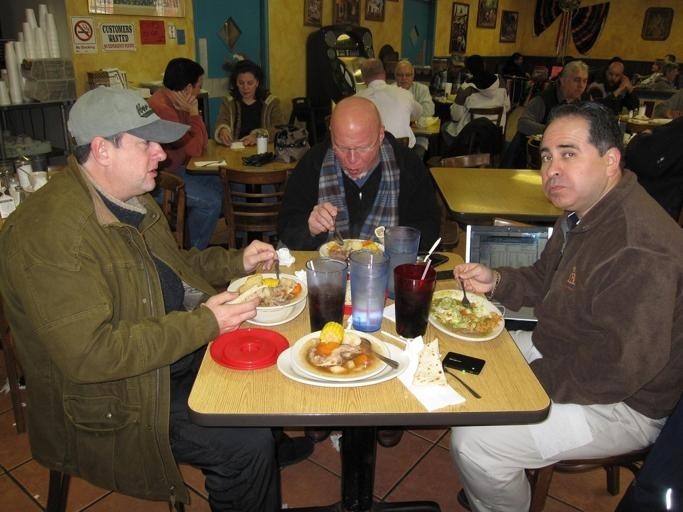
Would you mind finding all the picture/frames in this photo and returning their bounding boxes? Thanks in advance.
[332,0,362,26]
[449,2,470,55]
[303,0,324,27]
[476,0,499,29]
[499,10,519,43]
[364,0,385,22]
[642,7,674,41]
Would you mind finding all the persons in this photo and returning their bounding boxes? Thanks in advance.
[390,62,435,161]
[278,95,440,448]
[653,63,679,89]
[353,58,424,149]
[448,103,683,511]
[586,57,639,115]
[502,53,524,100]
[147,56,223,250]
[625,117,683,227]
[641,59,665,84]
[517,62,588,145]
[0,83,313,512]
[214,60,283,247]
[430,54,468,96]
[653,87,683,117]
[441,55,512,157]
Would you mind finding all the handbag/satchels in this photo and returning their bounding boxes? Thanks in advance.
[272,107,311,164]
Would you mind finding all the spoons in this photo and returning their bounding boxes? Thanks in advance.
[360,337,399,369]
[201,159,224,167]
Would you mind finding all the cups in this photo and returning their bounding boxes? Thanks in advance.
[383,226,421,300]
[305,257,347,333]
[393,264,436,340]
[349,248,391,333]
[203,139,216,162]
[256,133,268,155]
[638,103,646,117]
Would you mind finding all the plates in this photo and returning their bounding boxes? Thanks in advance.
[290,329,391,382]
[319,238,385,266]
[276,343,410,388]
[430,289,505,341]
[246,298,307,326]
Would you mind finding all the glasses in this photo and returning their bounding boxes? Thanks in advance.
[330,130,380,152]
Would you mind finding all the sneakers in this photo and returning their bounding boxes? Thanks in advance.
[377,430,403,447]
[458,489,468,510]
[304,426,332,439]
[277,437,312,465]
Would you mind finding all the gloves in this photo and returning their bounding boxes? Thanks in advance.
[241,152,274,166]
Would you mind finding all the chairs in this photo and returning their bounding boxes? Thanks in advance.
[441,152,492,167]
[217,166,291,248]
[155,169,186,250]
[469,105,504,131]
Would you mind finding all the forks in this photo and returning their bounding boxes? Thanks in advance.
[458,276,471,309]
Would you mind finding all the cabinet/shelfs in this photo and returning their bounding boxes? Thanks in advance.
[0,92,74,196]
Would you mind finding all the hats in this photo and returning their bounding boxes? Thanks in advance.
[67,85,191,144]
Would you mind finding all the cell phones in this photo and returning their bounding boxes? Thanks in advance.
[443,352,485,375]
[429,253,450,268]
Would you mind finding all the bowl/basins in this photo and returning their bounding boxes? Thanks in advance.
[27,171,47,190]
[227,273,308,323]
